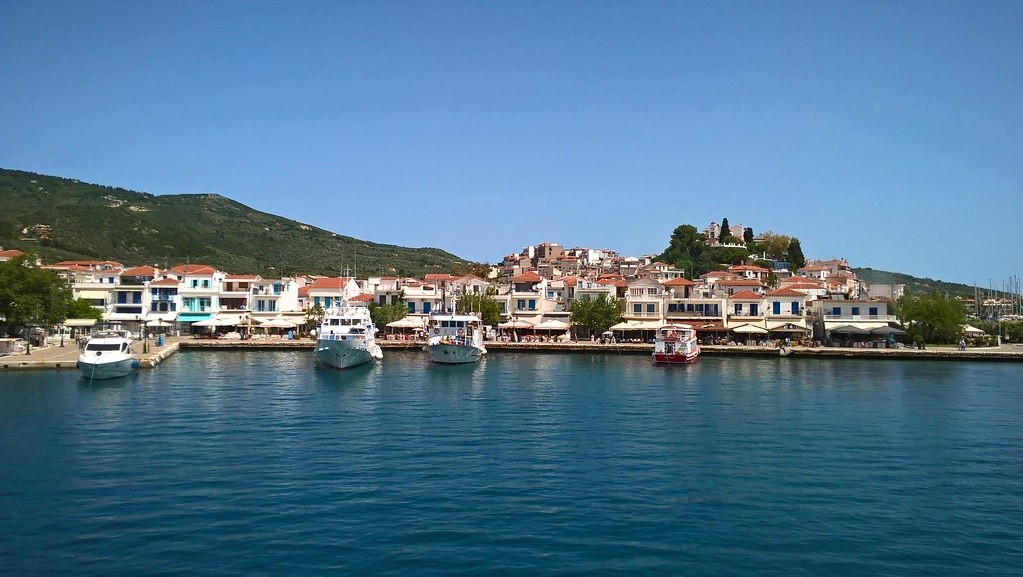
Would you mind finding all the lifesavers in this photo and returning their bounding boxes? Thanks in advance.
[457,328,464,334]
[434,328,440,334]
[670,332,678,340]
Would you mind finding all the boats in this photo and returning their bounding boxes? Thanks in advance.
[314,264,383,368]
[422,285,488,364]
[653,316,701,364]
[77,322,140,380]
